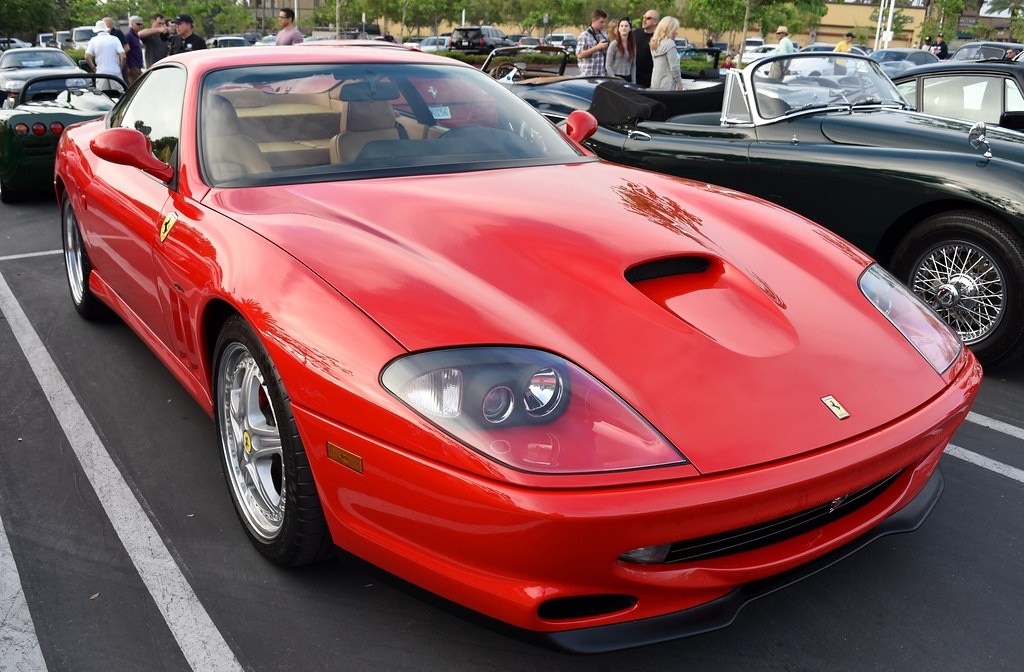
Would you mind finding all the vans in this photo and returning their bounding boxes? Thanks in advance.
[545,34,576,52]
[36,26,97,49]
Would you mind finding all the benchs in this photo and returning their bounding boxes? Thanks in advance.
[217,92,345,170]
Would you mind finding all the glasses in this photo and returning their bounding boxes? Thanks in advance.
[132,23,142,26]
[643,16,656,19]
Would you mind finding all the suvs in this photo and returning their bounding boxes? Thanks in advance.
[506,35,543,55]
[450,27,517,55]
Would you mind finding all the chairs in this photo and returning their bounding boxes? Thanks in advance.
[43,57,61,66]
[204,95,276,182]
[327,101,414,167]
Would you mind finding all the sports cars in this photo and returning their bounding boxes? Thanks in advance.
[483,52,1024,376]
[54,46,982,654]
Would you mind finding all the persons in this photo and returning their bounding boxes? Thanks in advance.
[1006,48,1016,60]
[85,12,206,94]
[384,31,394,42]
[928,33,948,60]
[275,8,304,45]
[768,26,794,81]
[720,55,735,69]
[575,9,683,92]
[833,33,856,75]
[919,36,932,51]
[706,35,715,63]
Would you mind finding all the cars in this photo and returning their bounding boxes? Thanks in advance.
[355,36,449,53]
[674,38,737,60]
[740,37,1023,134]
[0,38,129,202]
[204,33,277,47]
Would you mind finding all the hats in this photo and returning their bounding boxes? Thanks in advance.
[170,14,192,23]
[845,32,857,38]
[925,36,931,41]
[91,20,111,33]
[775,26,788,36]
[937,34,943,37]
